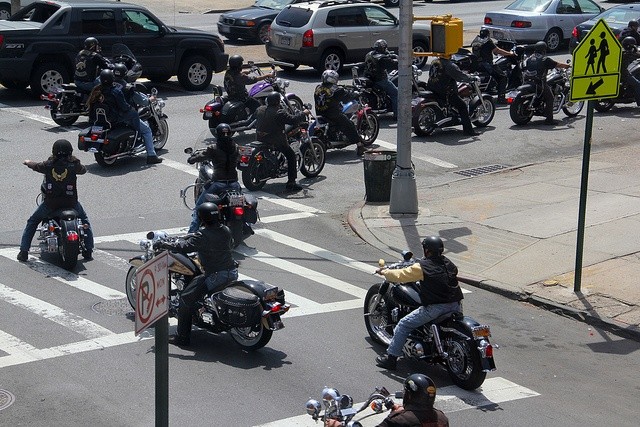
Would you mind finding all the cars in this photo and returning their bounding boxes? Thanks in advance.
[481,0,606,53]
[218,0,309,44]
[569,2,640,56]
[0,0,42,20]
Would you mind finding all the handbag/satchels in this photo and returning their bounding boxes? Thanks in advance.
[242,194,260,224]
[216,286,262,327]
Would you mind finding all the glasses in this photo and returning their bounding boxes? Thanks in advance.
[221,132,230,135]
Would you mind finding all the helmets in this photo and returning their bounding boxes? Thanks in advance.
[480,28,490,38]
[100,69,114,84]
[114,65,128,78]
[403,373,436,405]
[421,235,444,254]
[215,123,232,137]
[267,91,280,106]
[52,139,73,155]
[197,202,220,221]
[535,41,547,52]
[321,70,340,84]
[374,39,387,52]
[229,54,244,68]
[84,37,99,52]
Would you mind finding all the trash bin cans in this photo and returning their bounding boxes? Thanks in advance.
[362,150,397,203]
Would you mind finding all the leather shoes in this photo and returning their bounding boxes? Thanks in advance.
[464,131,482,136]
[375,355,397,370]
[18,251,28,261]
[168,335,190,347]
[357,146,373,156]
[148,156,162,162]
[287,181,302,191]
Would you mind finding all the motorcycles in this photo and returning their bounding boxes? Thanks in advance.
[303,84,379,159]
[200,60,304,140]
[364,251,500,391]
[454,44,528,98]
[505,59,584,125]
[237,102,327,191]
[306,385,404,427]
[77,87,169,169]
[23,162,90,268]
[125,231,291,355]
[353,54,427,120]
[412,71,496,137]
[593,57,640,112]
[40,43,150,127]
[180,147,260,249]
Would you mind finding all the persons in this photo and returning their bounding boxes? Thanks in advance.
[152,202,240,348]
[620,36,640,106]
[325,372,451,426]
[524,40,571,125]
[223,54,273,132]
[314,69,373,157]
[184,122,242,234]
[112,63,162,163]
[618,19,640,46]
[375,236,465,369]
[85,69,143,149]
[73,37,115,92]
[16,139,94,262]
[363,37,398,120]
[427,53,482,136]
[470,25,515,104]
[253,91,310,192]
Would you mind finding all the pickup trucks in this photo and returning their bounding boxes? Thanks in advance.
[0,0,229,101]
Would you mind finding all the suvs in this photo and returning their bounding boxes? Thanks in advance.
[266,0,433,77]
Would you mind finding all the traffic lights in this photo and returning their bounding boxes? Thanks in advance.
[430,22,458,54]
